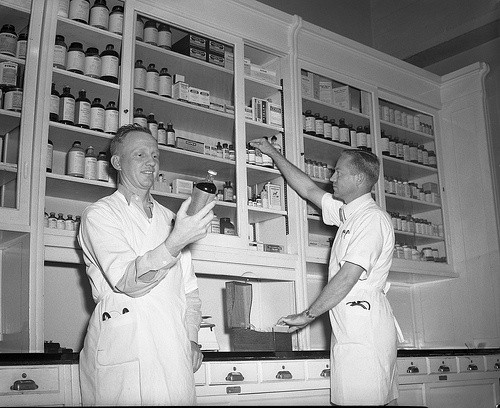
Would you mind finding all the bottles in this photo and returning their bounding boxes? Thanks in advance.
[50,83,119,135]
[134,59,148,91]
[392,240,447,264]
[66,141,118,185]
[215,141,236,160]
[246,135,282,169]
[53,35,119,84]
[46,139,54,173]
[186,169,236,236]
[387,210,444,237]
[142,21,159,46]
[0,23,18,57]
[301,109,372,153]
[0,61,23,113]
[134,108,176,147]
[15,33,28,60]
[135,16,144,41]
[58,0,124,35]
[44,208,81,231]
[159,25,172,50]
[146,64,160,95]
[159,68,172,98]
[381,128,437,169]
[247,189,268,209]
[383,175,433,204]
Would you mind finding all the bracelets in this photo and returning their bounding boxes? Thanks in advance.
[305,309,316,319]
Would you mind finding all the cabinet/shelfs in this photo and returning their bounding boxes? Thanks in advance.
[303,17,456,345]
[0,347,500,408]
[38,0,301,353]
[0,0,47,353]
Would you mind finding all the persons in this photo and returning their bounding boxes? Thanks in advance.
[77,124,216,407]
[248,138,404,405]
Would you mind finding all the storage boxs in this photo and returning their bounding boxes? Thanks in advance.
[225,283,293,353]
[300,70,354,111]
[243,97,282,126]
[173,74,211,108]
[265,183,282,212]
[176,137,203,153]
